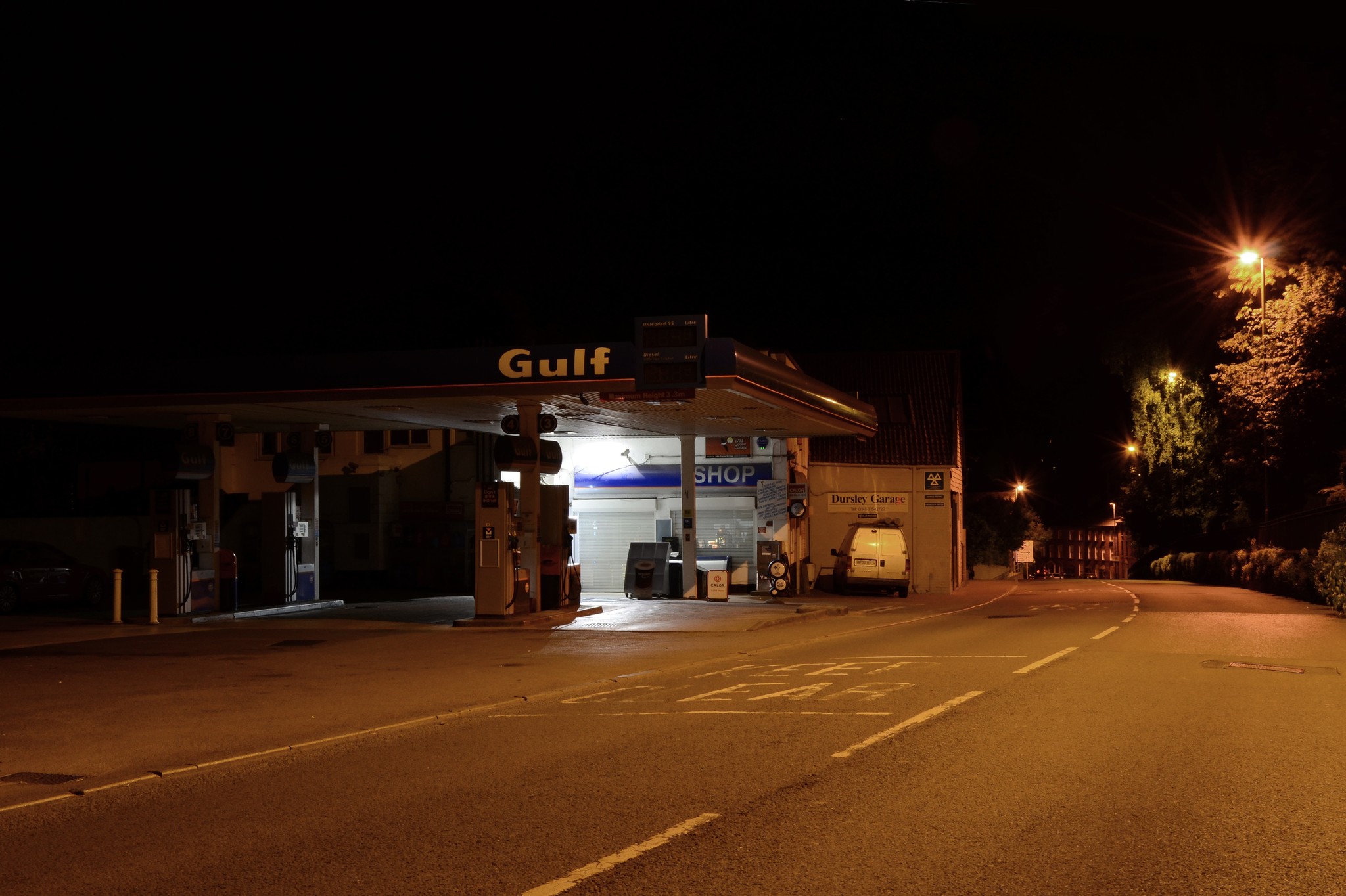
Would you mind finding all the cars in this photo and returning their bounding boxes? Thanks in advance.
[1049,572,1064,579]
[1080,573,1097,579]
[0,539,109,612]
[1029,572,1046,580]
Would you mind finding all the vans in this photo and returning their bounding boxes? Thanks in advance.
[830,523,911,598]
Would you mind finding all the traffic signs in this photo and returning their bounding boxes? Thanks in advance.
[1015,540,1034,562]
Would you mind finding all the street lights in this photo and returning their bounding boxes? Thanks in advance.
[1109,502,1117,579]
